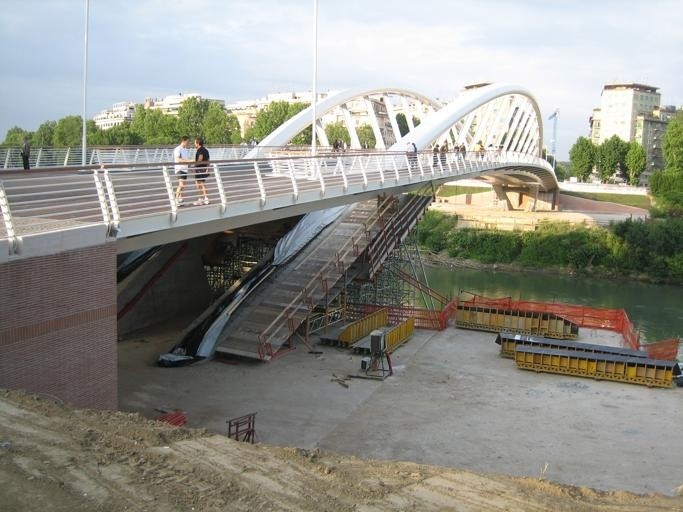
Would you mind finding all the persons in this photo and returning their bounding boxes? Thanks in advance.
[173,136,209,207]
[406,142,418,169]
[239,138,258,155]
[432,139,504,168]
[363,140,369,149]
[19,136,29,171]
[331,138,347,152]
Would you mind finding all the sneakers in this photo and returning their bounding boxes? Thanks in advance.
[176,198,184,206]
[193,198,209,206]
